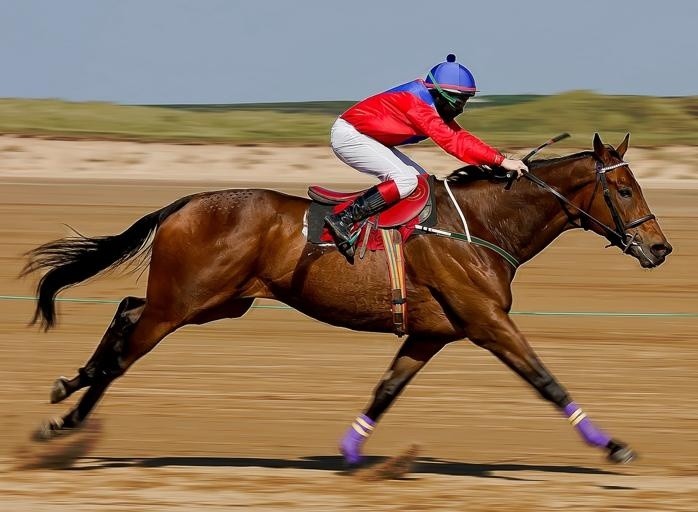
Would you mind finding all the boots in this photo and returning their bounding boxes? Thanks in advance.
[324,185,386,257]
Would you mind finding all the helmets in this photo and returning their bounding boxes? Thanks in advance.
[425,54,478,94]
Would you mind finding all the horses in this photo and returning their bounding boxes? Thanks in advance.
[15,134,674,472]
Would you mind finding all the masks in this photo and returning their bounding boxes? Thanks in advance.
[435,96,464,123]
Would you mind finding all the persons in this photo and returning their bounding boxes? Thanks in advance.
[323,62,529,243]
